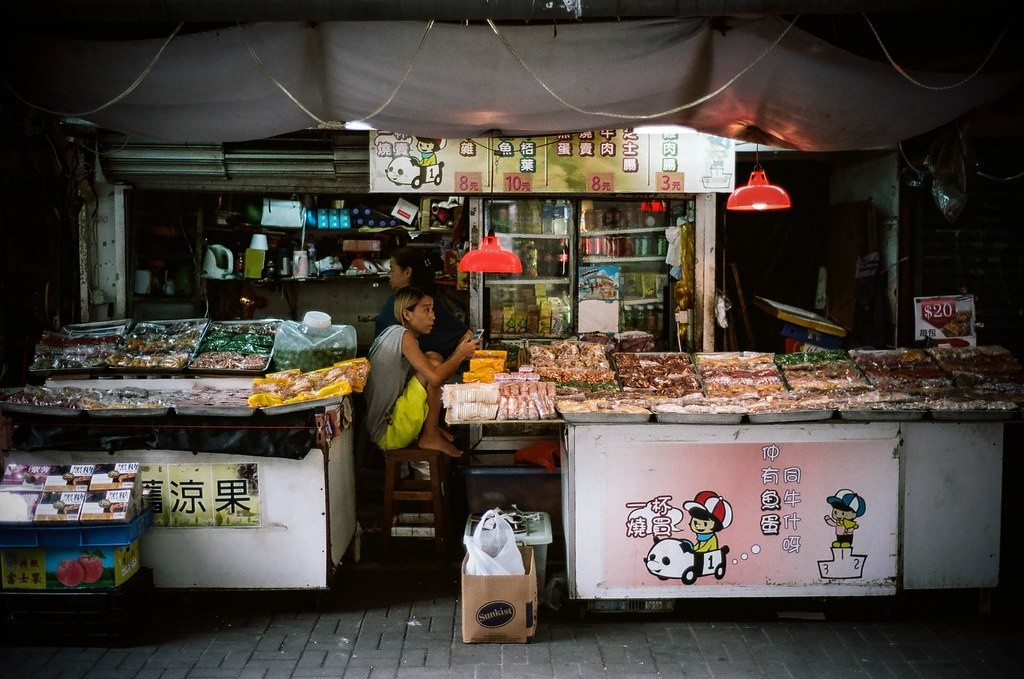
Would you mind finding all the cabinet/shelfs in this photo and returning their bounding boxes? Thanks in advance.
[558,422,1004,617]
[0,399,360,595]
[205,223,455,284]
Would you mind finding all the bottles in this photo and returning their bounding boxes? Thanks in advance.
[351,208,396,228]
[489,200,573,279]
[234,252,245,276]
[624,305,664,332]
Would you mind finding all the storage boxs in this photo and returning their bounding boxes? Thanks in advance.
[461,546,537,643]
[463,511,552,594]
[0,500,156,591]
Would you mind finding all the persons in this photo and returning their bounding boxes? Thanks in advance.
[364,287,480,458]
[374,248,470,372]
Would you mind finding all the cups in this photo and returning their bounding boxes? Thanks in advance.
[292,250,308,278]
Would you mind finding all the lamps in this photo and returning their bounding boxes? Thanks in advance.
[458,138,522,273]
[727,144,790,211]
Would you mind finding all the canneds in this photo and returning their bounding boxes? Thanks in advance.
[582,209,668,257]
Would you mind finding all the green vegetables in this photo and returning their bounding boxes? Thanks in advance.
[556,379,618,391]
[775,348,845,366]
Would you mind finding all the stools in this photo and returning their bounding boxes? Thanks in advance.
[381,440,446,553]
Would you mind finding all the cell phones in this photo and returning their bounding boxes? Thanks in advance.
[469,329,485,343]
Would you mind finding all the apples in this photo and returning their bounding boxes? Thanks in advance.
[57,550,107,586]
